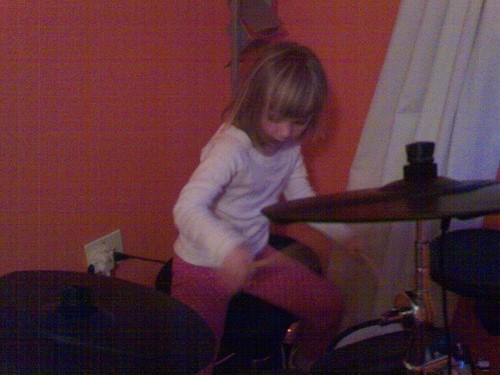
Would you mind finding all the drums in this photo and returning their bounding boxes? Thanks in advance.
[334,318,434,350]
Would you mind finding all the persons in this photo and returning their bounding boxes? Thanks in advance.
[171,40,347,375]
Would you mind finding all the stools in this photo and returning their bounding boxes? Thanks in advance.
[155,234,321,375]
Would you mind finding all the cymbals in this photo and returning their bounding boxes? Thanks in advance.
[311,326,500,375]
[260,175,500,224]
[0,268,215,375]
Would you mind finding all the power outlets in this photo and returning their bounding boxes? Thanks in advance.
[84,229,124,273]
